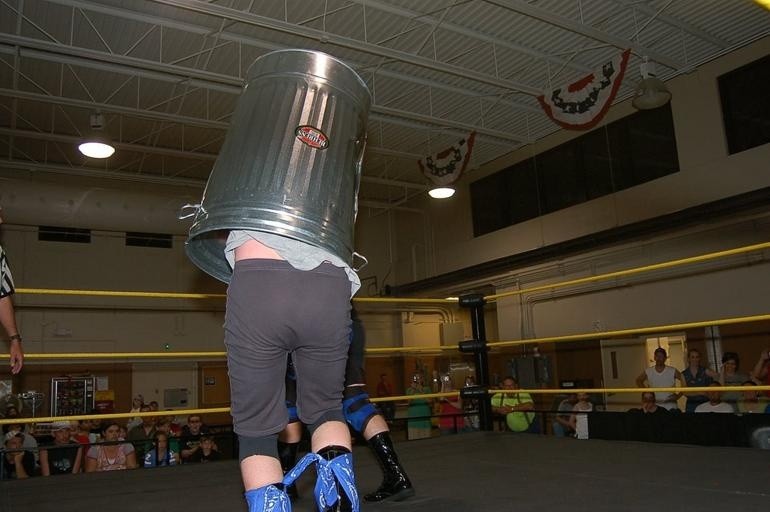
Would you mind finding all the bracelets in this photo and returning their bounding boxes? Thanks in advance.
[7,334,23,343]
[511,406,514,412]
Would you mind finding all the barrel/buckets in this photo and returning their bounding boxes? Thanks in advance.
[177,47,376,286]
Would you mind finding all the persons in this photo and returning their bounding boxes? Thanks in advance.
[376,373,397,425]
[405,369,434,441]
[0,207,25,376]
[636,347,682,415]
[463,375,482,432]
[638,392,674,416]
[491,376,541,435]
[681,349,719,414]
[553,393,577,438]
[694,381,734,413]
[436,373,465,435]
[568,392,596,440]
[714,351,750,413]
[752,348,770,397]
[275,300,414,504]
[1,394,220,479]
[221,222,360,512]
[736,380,770,414]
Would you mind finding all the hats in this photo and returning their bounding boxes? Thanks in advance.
[3,430,25,444]
[51,421,71,431]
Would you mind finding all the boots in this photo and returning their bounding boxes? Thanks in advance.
[363,431,415,503]
[275,442,299,501]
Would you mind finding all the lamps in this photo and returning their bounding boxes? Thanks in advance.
[633,56,672,110]
[76,107,117,160]
